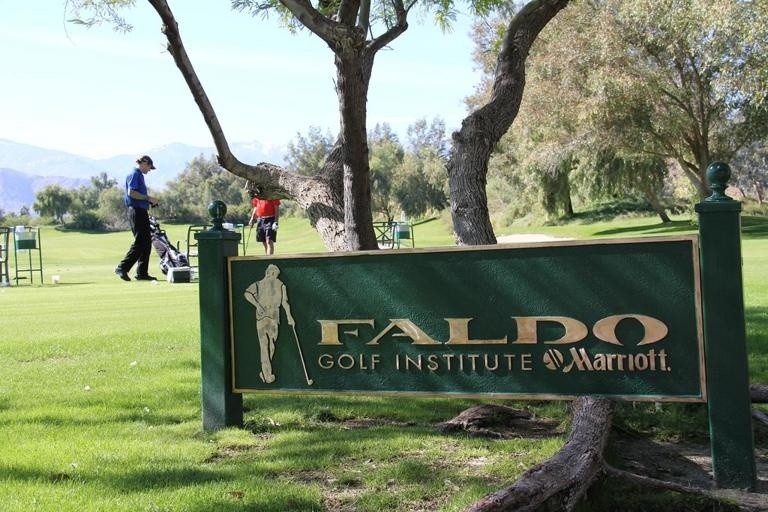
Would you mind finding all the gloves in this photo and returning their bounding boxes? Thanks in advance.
[271,222,279,231]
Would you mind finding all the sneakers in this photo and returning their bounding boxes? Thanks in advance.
[115,268,131,281]
[135,275,158,280]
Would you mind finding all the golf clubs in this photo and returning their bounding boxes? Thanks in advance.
[291,324,314,386]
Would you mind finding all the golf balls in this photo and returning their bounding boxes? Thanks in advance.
[131,361,137,368]
[151,279,158,285]
[85,385,90,391]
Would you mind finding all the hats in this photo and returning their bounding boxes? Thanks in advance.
[135,155,156,170]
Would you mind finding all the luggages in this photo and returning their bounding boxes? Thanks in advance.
[148,216,191,274]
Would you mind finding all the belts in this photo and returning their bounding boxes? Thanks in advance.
[260,215,272,219]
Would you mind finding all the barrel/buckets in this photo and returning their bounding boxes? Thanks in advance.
[16,231,37,249]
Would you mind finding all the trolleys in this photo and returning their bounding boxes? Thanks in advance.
[149,206,188,274]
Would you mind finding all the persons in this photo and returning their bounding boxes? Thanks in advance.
[249,197,281,255]
[115,155,159,281]
[244,263,295,384]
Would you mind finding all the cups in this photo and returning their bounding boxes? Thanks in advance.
[52,275,59,284]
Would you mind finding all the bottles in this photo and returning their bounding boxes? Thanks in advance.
[401,210,406,222]
